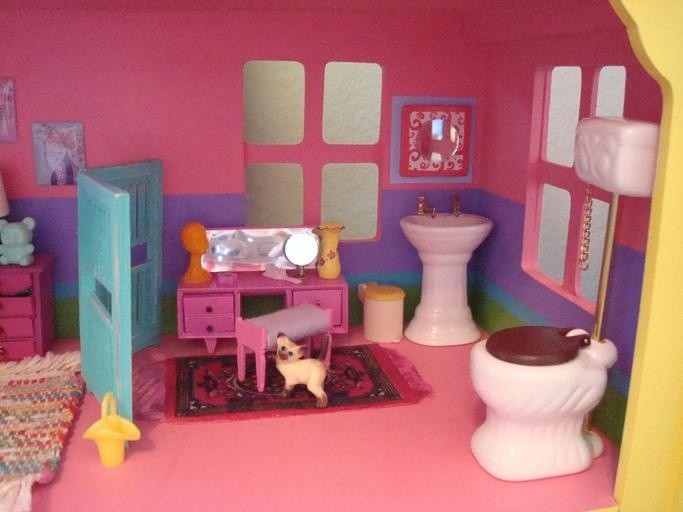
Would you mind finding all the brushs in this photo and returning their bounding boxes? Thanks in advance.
[262,263,302,284]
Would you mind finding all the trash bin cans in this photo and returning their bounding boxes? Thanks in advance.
[363,286,405,343]
